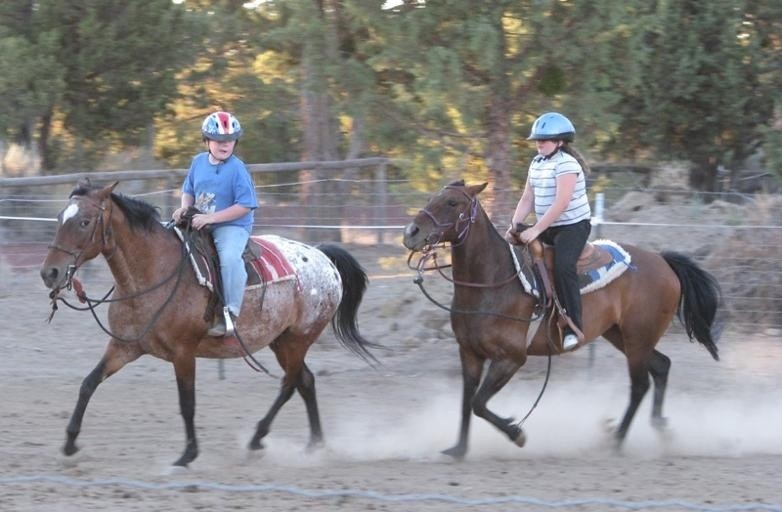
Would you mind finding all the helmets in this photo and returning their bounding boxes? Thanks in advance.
[202,112,242,142]
[526,113,576,141]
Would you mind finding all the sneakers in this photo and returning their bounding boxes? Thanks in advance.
[563,335,579,350]
[209,312,237,336]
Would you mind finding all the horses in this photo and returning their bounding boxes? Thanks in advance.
[39,174,396,478]
[401,177,725,466]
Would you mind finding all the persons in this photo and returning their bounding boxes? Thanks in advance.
[170,110,259,338]
[501,111,593,351]
